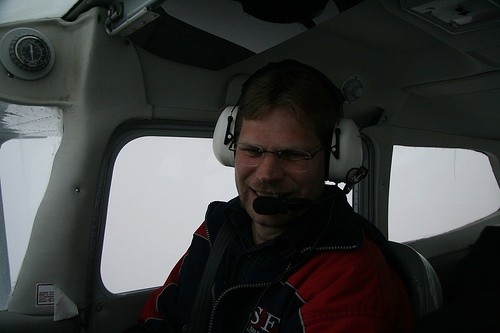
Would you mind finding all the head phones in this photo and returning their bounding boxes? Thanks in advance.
[211,58,369,184]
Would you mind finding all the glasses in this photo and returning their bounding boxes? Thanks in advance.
[228,136,327,172]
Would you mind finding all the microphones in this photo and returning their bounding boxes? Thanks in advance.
[253,183,351,216]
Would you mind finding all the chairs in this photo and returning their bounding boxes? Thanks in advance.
[387,241,442,323]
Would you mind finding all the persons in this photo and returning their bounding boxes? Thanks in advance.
[138,59,416,333]
